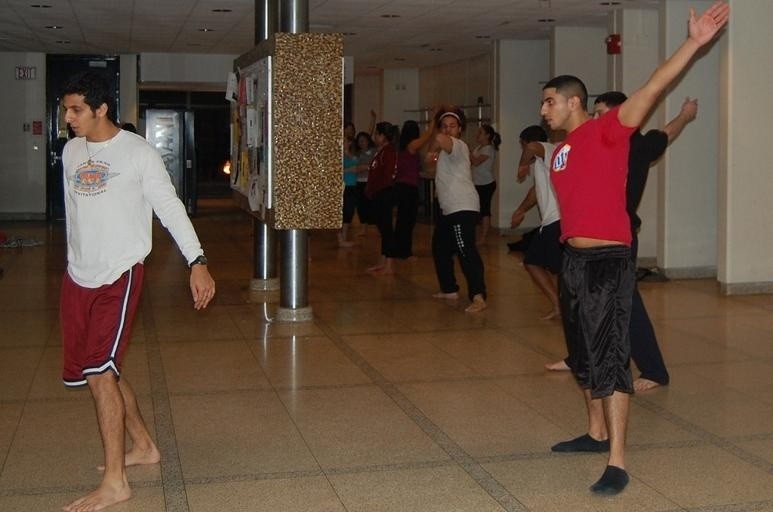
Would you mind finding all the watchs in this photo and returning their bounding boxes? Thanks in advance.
[189,254,209,267]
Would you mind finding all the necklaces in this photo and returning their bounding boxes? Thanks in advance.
[83,126,120,165]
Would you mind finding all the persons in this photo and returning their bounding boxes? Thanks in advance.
[537,0,731,497]
[467,123,501,246]
[394,105,439,262]
[333,135,361,249]
[509,124,561,321]
[423,104,488,314]
[354,131,379,238]
[344,106,377,142]
[58,71,219,511]
[363,120,399,276]
[543,90,699,391]
